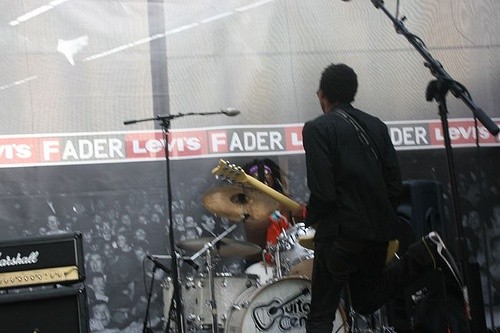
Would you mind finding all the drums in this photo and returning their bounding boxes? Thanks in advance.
[220,276,349,333]
[272,222,315,278]
[160,271,202,332]
[194,271,259,327]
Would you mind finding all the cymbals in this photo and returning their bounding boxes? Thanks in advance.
[203,187,280,220]
[176,236,262,256]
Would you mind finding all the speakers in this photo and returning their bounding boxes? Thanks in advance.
[395,179,446,259]
[0,282,90,333]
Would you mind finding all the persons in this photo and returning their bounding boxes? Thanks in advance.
[240,158,304,266]
[292,64,404,333]
[0,165,500,333]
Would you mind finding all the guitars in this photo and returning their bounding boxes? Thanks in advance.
[211,158,316,251]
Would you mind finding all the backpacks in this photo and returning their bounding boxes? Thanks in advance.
[383,232,470,333]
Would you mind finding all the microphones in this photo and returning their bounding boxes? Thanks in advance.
[221,108,241,116]
[150,257,171,274]
[175,247,199,270]
